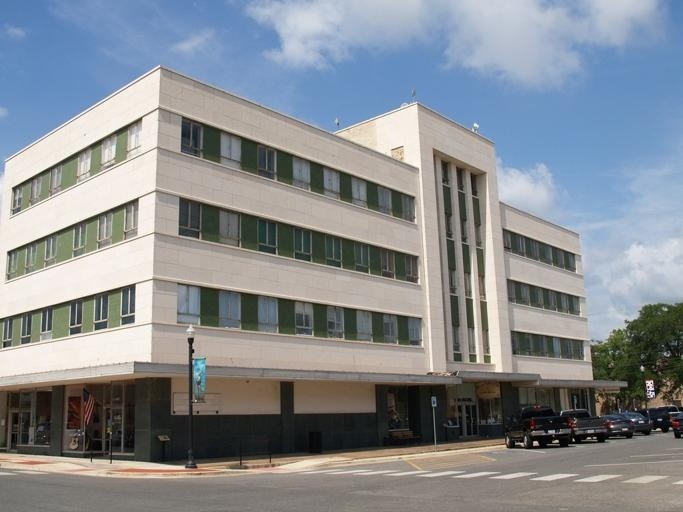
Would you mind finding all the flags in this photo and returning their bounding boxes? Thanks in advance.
[82,388,95,426]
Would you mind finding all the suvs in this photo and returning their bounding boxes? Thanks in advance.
[35,422,92,452]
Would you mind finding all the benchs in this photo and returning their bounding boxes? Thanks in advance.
[390,431,421,446]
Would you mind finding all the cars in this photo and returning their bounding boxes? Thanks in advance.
[502,403,683,449]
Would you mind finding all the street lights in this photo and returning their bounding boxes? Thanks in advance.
[183,325,198,469]
[638,363,649,417]
